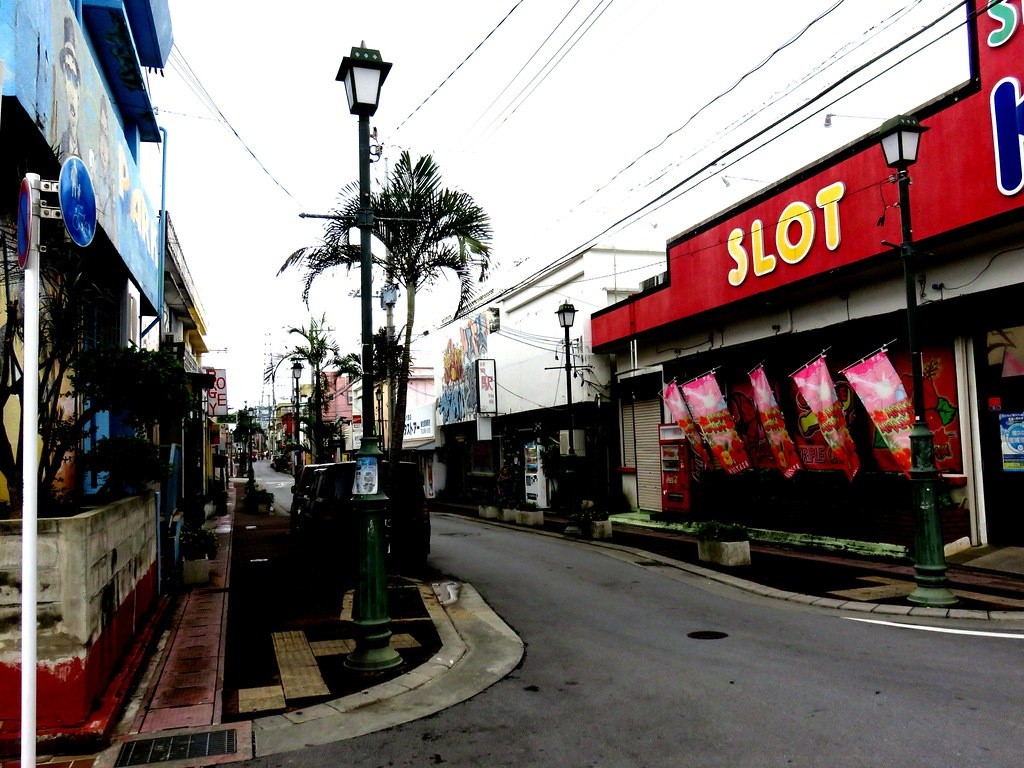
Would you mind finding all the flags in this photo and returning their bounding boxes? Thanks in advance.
[682,373,751,474]
[747,366,803,478]
[661,383,713,470]
[793,358,861,482]
[846,353,941,480]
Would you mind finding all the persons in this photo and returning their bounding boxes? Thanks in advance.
[497,467,515,507]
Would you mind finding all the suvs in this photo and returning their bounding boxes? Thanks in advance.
[290,460,432,571]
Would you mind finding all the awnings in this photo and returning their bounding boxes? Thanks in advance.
[402,438,435,451]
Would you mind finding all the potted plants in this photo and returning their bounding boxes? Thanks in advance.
[251,489,275,512]
[577,499,612,538]
[693,520,751,566]
[478,498,544,525]
[177,526,221,583]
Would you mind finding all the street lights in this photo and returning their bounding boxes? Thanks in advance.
[337,39,405,680]
[876,114,961,609]
[556,299,578,458]
[291,359,303,484]
